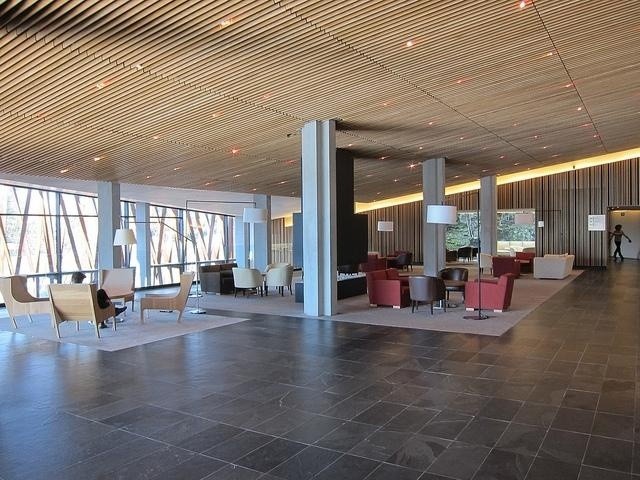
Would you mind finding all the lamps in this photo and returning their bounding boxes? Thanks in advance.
[183,200,268,299]
[113,216,206,315]
[377,221,395,270]
[514,208,564,255]
[426,191,490,322]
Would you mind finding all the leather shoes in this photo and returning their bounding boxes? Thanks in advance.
[115,306,127,318]
[100,324,108,328]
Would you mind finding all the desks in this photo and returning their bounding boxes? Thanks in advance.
[432,300,446,310]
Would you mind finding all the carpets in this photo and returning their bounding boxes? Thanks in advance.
[136,263,586,338]
[1,309,249,354]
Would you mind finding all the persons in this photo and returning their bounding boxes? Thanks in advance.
[70,271,127,328]
[609,224,631,262]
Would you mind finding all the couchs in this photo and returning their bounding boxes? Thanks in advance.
[199,263,243,295]
[366,270,421,309]
[464,273,515,313]
[492,254,574,280]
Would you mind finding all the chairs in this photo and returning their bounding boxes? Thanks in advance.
[358,251,413,272]
[446,246,481,264]
[477,252,493,275]
[98,266,137,317]
[1,275,55,331]
[232,259,294,298]
[437,268,468,304]
[47,281,118,339]
[138,270,195,324]
[408,275,446,315]
[522,247,535,253]
